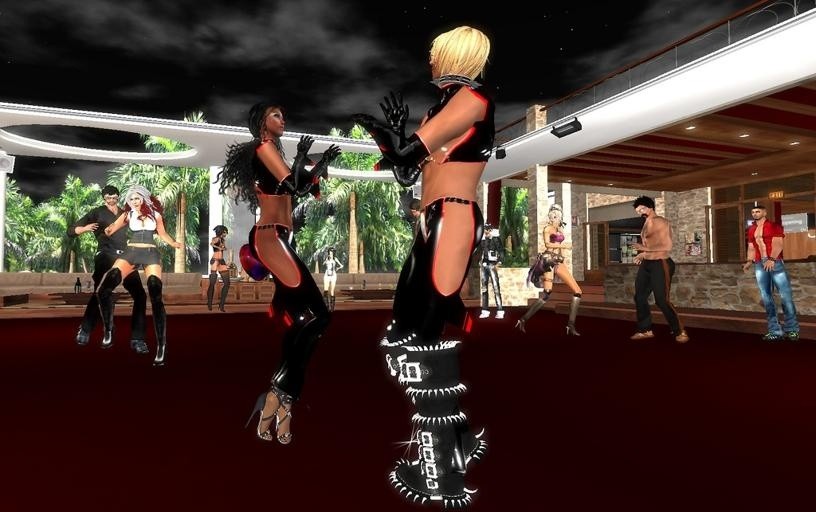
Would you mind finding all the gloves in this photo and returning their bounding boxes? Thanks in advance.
[349,91,431,189]
[278,135,342,197]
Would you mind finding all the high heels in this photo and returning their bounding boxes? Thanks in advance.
[242,387,294,445]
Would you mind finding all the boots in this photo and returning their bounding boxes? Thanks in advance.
[146,275,168,367]
[566,296,581,337]
[95,267,123,350]
[515,297,546,333]
[218,270,230,312]
[323,291,336,313]
[207,274,217,311]
[379,319,489,506]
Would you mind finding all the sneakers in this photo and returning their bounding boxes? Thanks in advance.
[495,310,505,319]
[674,331,689,343]
[762,333,783,341]
[478,310,491,318]
[630,330,655,341]
[129,339,150,354]
[787,331,799,341]
[75,326,90,345]
